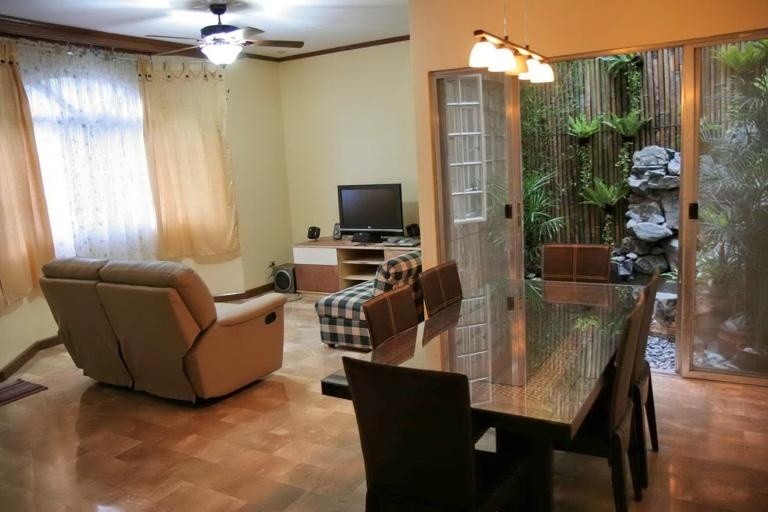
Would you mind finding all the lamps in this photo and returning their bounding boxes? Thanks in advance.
[466,37,502,71]
[199,24,246,69]
[535,61,553,85]
[523,55,538,82]
[488,48,517,74]
[509,51,528,79]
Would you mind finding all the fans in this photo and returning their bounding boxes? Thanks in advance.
[145,0,304,58]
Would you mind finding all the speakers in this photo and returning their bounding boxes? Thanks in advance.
[406,225,420,238]
[308,227,320,240]
[273,264,296,294]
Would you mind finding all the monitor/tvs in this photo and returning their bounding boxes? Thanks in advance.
[337,184,404,237]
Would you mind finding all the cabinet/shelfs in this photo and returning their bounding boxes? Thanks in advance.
[292,236,422,295]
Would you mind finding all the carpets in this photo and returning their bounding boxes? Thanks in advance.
[0,376,48,407]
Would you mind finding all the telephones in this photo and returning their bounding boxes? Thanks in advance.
[398,237,420,247]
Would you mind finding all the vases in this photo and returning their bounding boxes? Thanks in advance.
[716,324,755,359]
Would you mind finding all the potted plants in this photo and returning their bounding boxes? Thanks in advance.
[577,175,629,215]
[564,112,604,145]
[601,111,654,143]
[697,255,747,299]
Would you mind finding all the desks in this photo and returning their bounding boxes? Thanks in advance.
[320,278,647,512]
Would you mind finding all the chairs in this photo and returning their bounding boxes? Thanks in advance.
[416,261,462,319]
[629,266,662,491]
[340,354,524,511]
[361,285,418,348]
[539,243,612,286]
[552,288,645,512]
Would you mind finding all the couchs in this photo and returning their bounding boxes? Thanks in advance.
[95,258,287,407]
[313,249,421,354]
[37,255,133,405]
